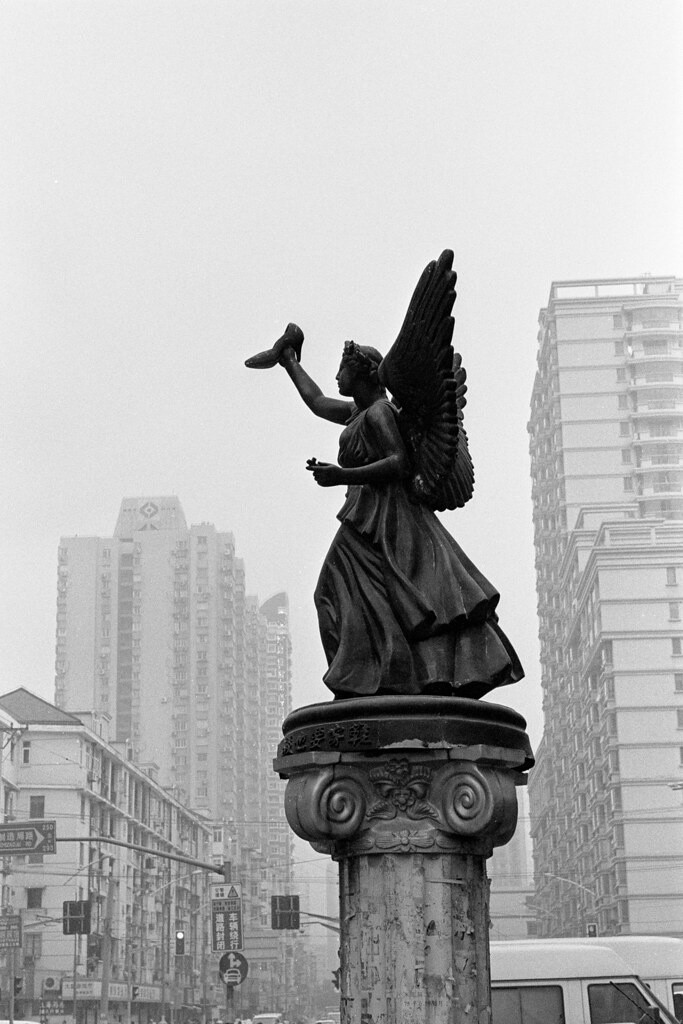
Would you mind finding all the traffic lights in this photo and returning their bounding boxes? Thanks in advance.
[175,930,185,955]
[131,985,138,1001]
[587,923,598,938]
[331,970,339,989]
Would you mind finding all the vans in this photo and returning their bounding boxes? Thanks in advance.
[489,937,683,1024]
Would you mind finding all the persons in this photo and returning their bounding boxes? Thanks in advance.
[279,341,525,701]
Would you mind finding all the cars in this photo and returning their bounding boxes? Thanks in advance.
[252,1013,282,1024]
